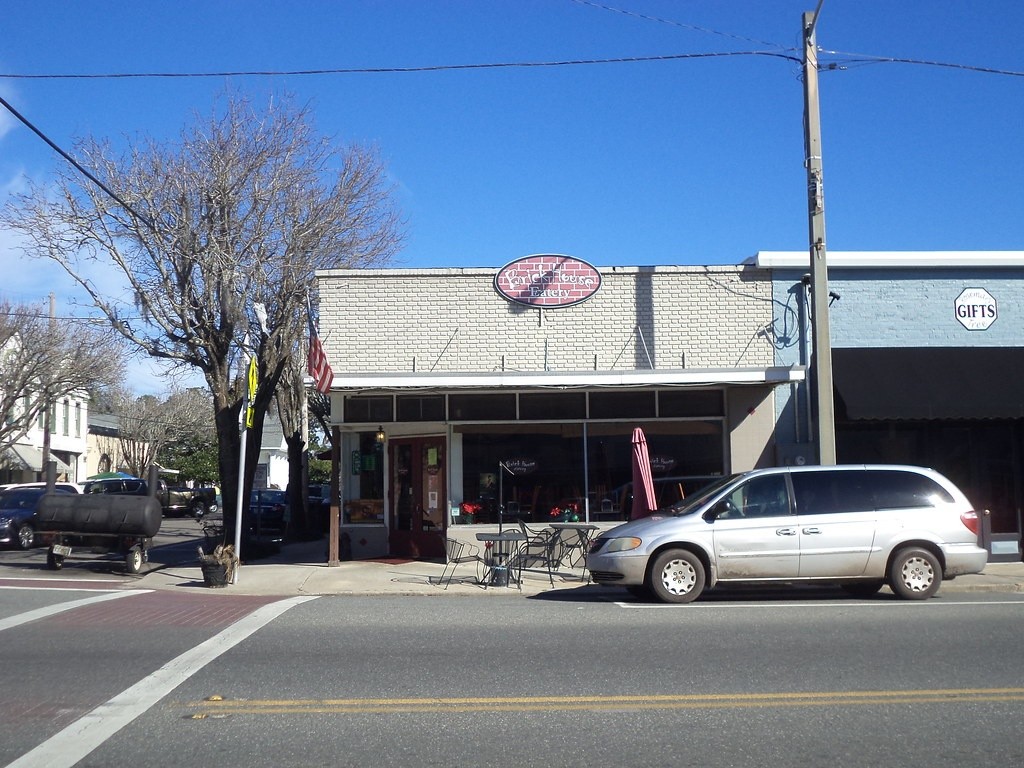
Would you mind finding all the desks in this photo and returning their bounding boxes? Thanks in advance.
[549,524,600,570]
[593,511,621,521]
[502,510,532,524]
[476,532,528,584]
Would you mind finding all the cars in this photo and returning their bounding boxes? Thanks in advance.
[168,486,218,513]
[247,488,286,530]
[0,486,71,551]
[0,481,84,494]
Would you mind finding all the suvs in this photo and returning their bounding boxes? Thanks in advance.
[585,463,989,604]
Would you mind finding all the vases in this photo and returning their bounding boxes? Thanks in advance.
[551,516,563,522]
[465,514,474,524]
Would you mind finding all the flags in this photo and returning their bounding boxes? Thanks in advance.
[308,307,334,395]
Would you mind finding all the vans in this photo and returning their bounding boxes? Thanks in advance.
[308,483,331,504]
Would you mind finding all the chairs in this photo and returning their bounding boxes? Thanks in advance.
[438,518,596,591]
[522,485,540,523]
[763,495,785,515]
[743,497,762,516]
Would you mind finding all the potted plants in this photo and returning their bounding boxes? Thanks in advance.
[196,544,238,590]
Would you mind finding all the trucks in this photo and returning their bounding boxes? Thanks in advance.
[30,460,164,575]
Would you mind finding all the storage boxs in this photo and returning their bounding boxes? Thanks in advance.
[344,499,384,525]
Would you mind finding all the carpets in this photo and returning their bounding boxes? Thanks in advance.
[363,555,434,565]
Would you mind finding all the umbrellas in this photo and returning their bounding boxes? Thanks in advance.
[631,428,656,518]
[833,346,1024,422]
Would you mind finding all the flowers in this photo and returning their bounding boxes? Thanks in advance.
[550,507,561,517]
[459,501,483,515]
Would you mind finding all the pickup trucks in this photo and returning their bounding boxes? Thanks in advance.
[145,479,213,518]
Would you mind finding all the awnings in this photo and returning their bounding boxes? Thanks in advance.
[12,444,74,473]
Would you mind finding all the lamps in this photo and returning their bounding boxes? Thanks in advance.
[375,425,386,451]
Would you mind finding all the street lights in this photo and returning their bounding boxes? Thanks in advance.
[41,316,108,479]
